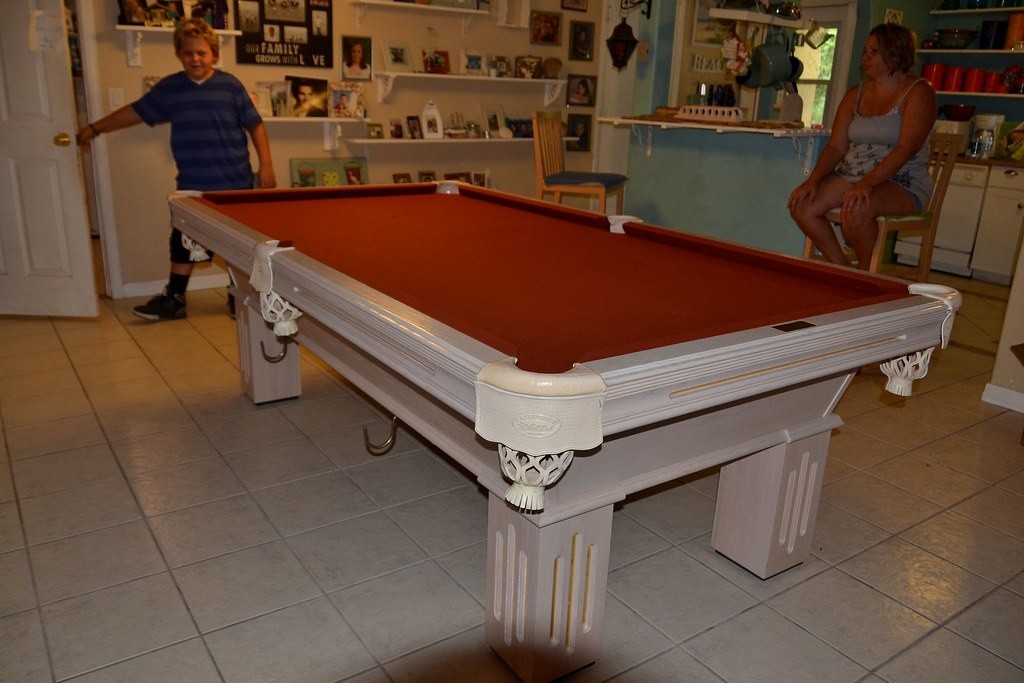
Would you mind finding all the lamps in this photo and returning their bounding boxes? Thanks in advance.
[607,6,639,71]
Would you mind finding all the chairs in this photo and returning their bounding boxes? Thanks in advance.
[804,131,963,284]
[531,110,626,215]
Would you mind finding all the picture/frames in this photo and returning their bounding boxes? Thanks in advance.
[246,0,598,187]
[692,0,739,48]
[884,7,903,24]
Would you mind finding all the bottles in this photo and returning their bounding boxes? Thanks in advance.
[695,81,736,106]
[968,129,994,159]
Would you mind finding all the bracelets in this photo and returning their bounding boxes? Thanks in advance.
[88,123,103,138]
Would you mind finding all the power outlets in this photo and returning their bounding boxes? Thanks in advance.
[108,87,126,111]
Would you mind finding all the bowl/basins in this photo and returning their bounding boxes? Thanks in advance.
[933,27,981,49]
[943,102,975,121]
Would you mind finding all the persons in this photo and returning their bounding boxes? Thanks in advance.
[289,82,324,116]
[568,78,593,105]
[342,38,371,80]
[334,93,348,114]
[509,123,534,138]
[787,22,940,268]
[158,0,213,26]
[567,120,589,150]
[573,31,590,56]
[77,19,276,321]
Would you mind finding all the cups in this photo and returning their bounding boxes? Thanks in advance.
[922,63,1007,94]
[941,0,1022,10]
[1004,14,1024,49]
[979,20,1007,50]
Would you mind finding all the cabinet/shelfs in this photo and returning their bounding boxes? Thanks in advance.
[914,7,1024,98]
[894,154,1024,415]
[115,0,579,144]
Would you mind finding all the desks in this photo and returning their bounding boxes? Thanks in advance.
[598,110,832,257]
[169,180,962,683]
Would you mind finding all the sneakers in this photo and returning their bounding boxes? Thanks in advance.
[226,285,236,318]
[133,285,187,319]
